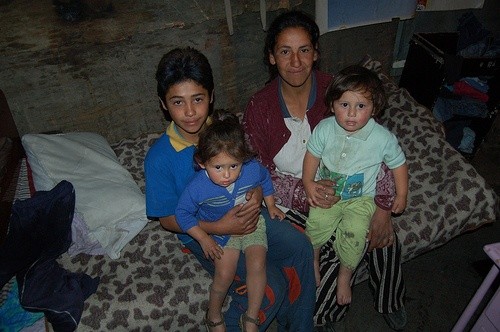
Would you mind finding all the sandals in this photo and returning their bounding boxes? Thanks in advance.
[239,310,260,332]
[203,310,227,332]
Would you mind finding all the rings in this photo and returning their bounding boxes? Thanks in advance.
[325,193,329,200]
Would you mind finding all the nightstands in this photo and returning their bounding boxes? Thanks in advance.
[397,31,500,161]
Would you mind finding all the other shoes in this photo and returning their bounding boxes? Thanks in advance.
[383,308,408,330]
[316,321,336,332]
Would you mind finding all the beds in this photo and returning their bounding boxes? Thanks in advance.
[0,73,500,332]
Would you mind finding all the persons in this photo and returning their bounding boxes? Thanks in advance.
[175,118,286,332]
[244,11,407,332]
[302,64,409,305]
[141,47,317,332]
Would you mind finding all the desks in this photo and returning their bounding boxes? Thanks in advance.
[452,243,500,332]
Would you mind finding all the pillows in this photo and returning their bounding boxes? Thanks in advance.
[16,133,150,260]
